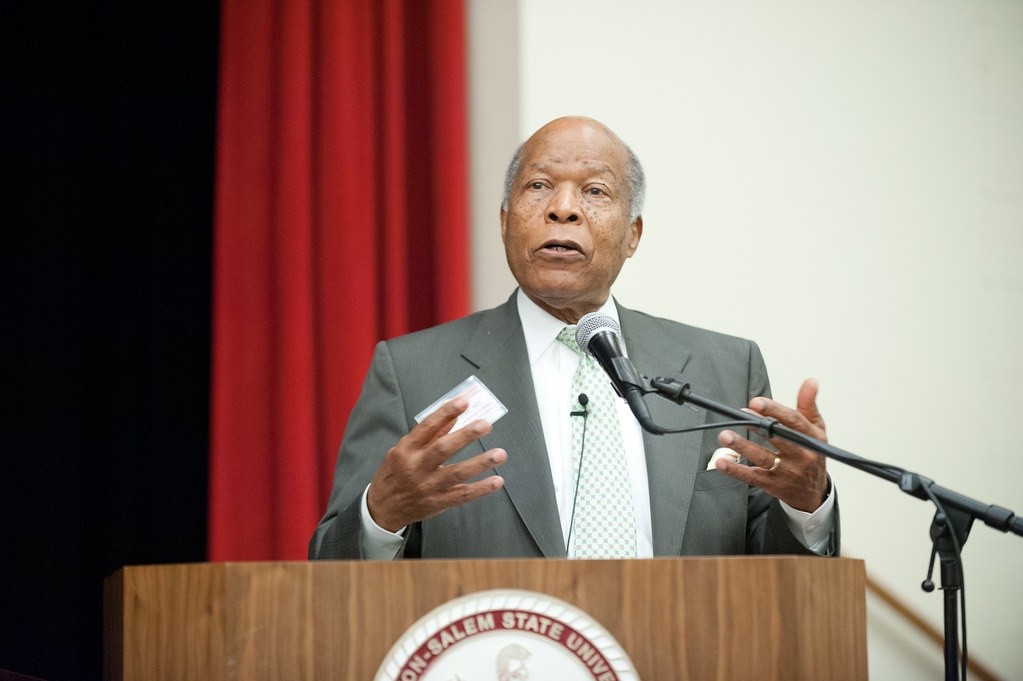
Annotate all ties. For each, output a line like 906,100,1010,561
557,325,637,560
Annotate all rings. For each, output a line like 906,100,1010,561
768,454,780,472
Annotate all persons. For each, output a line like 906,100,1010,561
309,117,842,559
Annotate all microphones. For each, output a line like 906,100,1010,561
576,312,664,434
570,393,588,417
